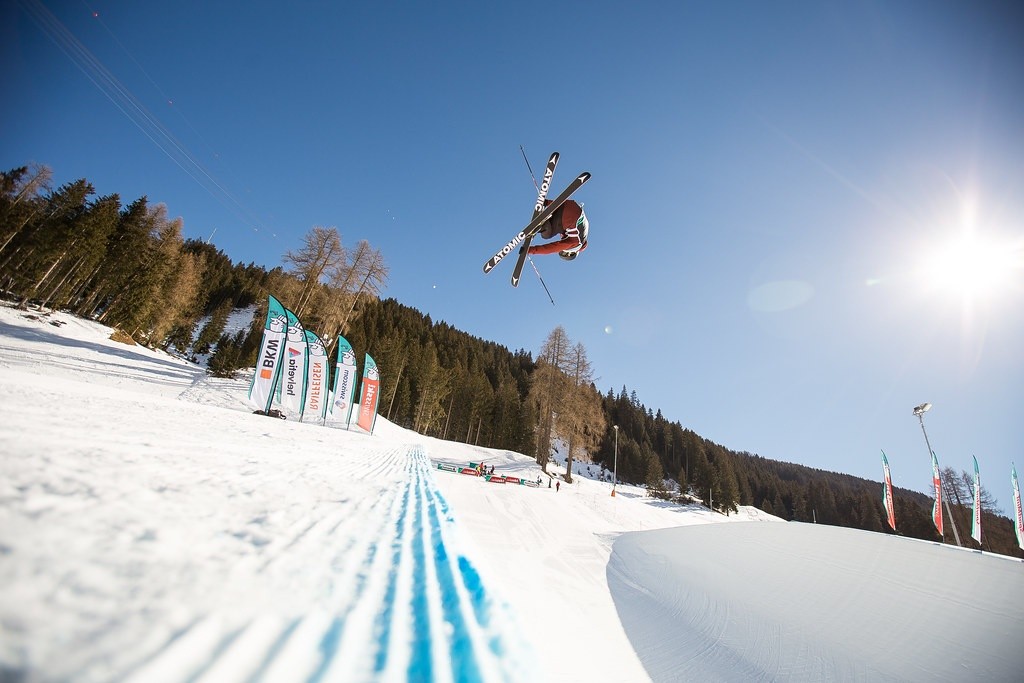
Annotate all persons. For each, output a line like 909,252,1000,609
519,200,589,260
537,475,560,492
476,462,495,477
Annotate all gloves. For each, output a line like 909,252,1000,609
519,247,524,254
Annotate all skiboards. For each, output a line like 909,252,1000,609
482,152,592,288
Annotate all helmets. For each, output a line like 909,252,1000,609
559,251,579,262
